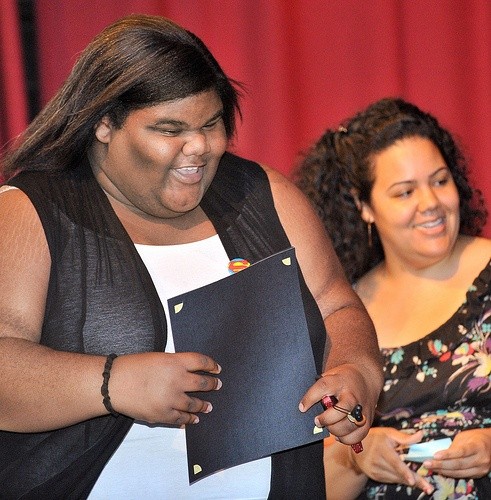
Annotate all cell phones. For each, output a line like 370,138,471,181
315,377,363,454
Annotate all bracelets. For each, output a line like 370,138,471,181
347,445,362,476
99,353,124,415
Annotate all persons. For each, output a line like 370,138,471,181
0,14,386,500
293,97,491,500
